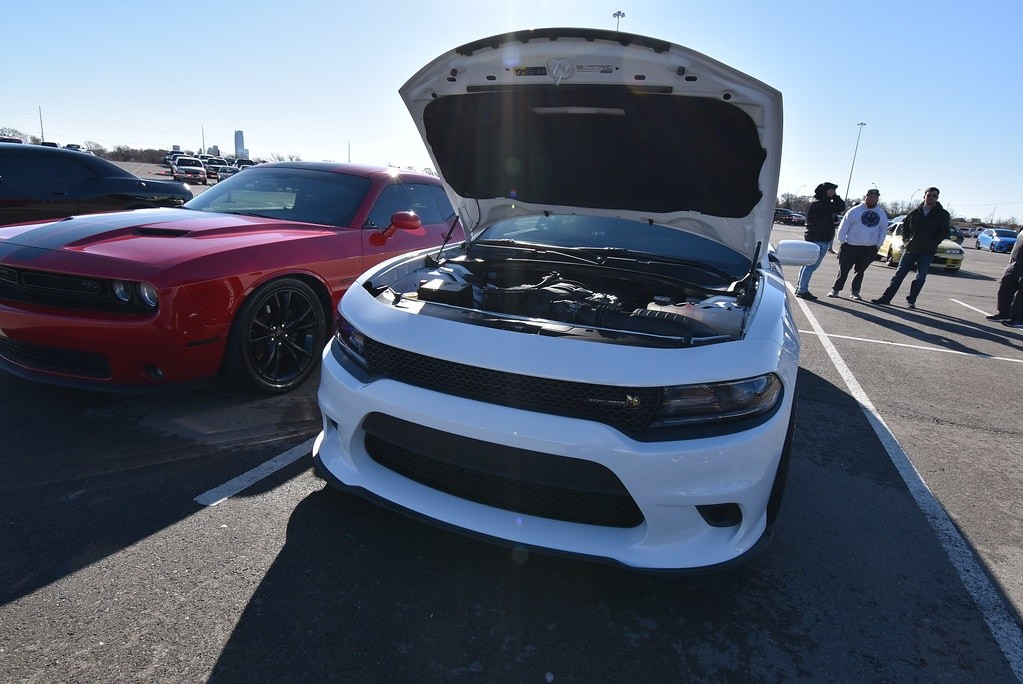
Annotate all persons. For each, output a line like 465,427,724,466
795,182,846,302
827,189,888,301
986,226,1023,328
871,187,952,308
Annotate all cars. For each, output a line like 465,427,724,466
774,209,807,225
0,138,22,143
215,155,255,170
64,144,86,152
0,159,467,399
164,150,191,173
41,142,60,148
975,229,1018,253
198,154,215,164
950,225,986,245
313,28,822,573
875,221,964,274
216,166,241,183
204,158,229,178
0,143,194,226
171,156,208,186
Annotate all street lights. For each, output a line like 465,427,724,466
845,122,866,203
612,11,625,31
909,189,921,206
872,182,877,189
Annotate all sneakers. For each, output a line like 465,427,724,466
985,313,1012,322
849,292,862,300
1000,319,1023,328
827,289,839,298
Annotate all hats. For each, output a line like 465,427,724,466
866,189,881,196
813,182,838,200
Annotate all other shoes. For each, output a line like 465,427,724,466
794,289,818,300
906,301,915,308
871,296,890,305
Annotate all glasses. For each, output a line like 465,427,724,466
927,194,938,197
